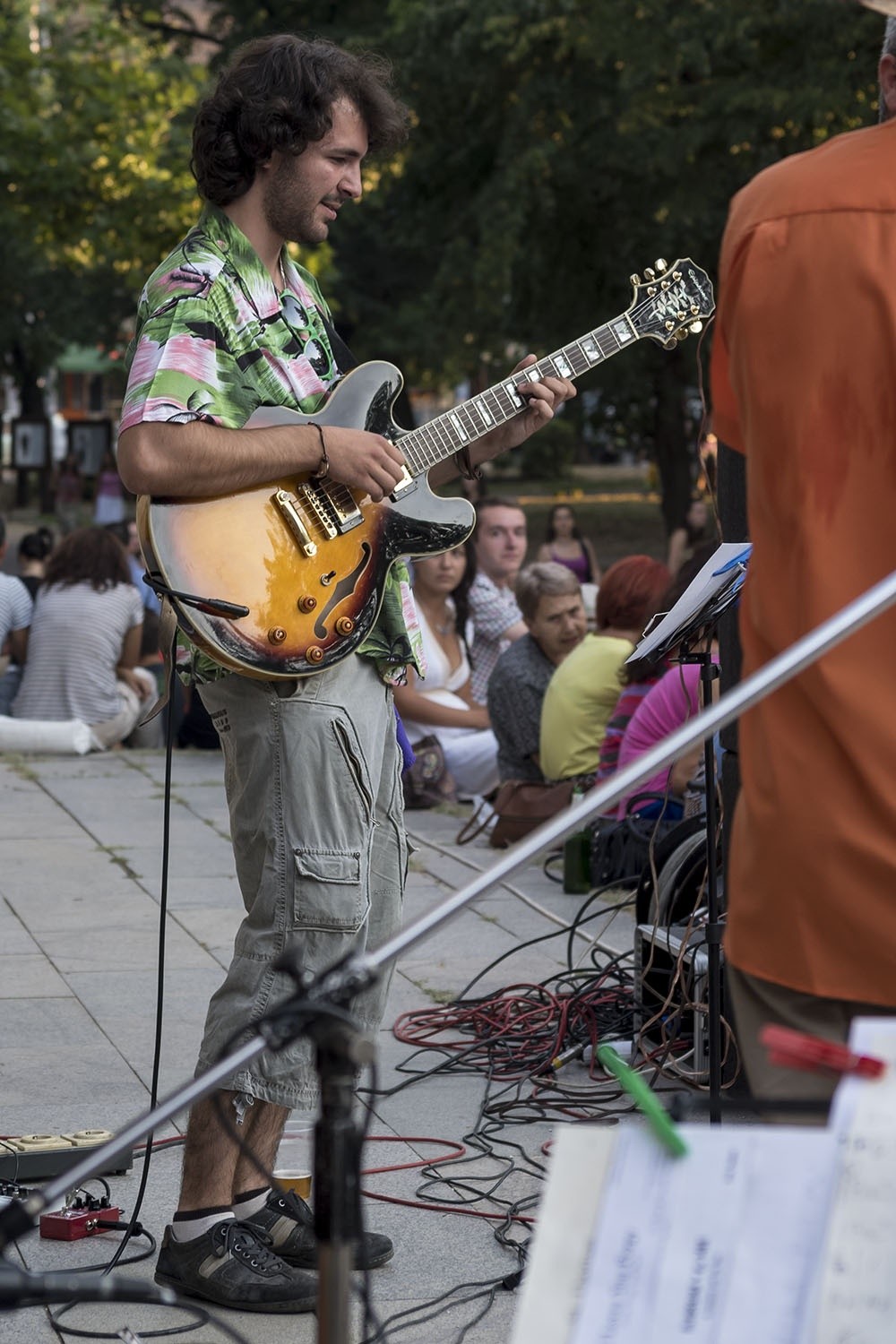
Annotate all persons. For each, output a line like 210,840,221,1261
397,497,721,888
115,37,577,1314
0,516,222,750
705,12,896,1122
11,441,129,523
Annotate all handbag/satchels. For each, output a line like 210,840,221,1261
404,717,460,809
457,781,574,849
591,792,723,890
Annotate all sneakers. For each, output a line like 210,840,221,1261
240,1187,395,1271
154,1218,320,1315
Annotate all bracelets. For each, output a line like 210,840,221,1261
305,419,331,487
455,445,482,485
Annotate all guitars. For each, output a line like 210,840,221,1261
135,258,716,682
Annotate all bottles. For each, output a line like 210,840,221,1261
564,783,590,894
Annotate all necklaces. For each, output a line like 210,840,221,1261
273,253,287,293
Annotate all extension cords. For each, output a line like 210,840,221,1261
0,1129,132,1182
